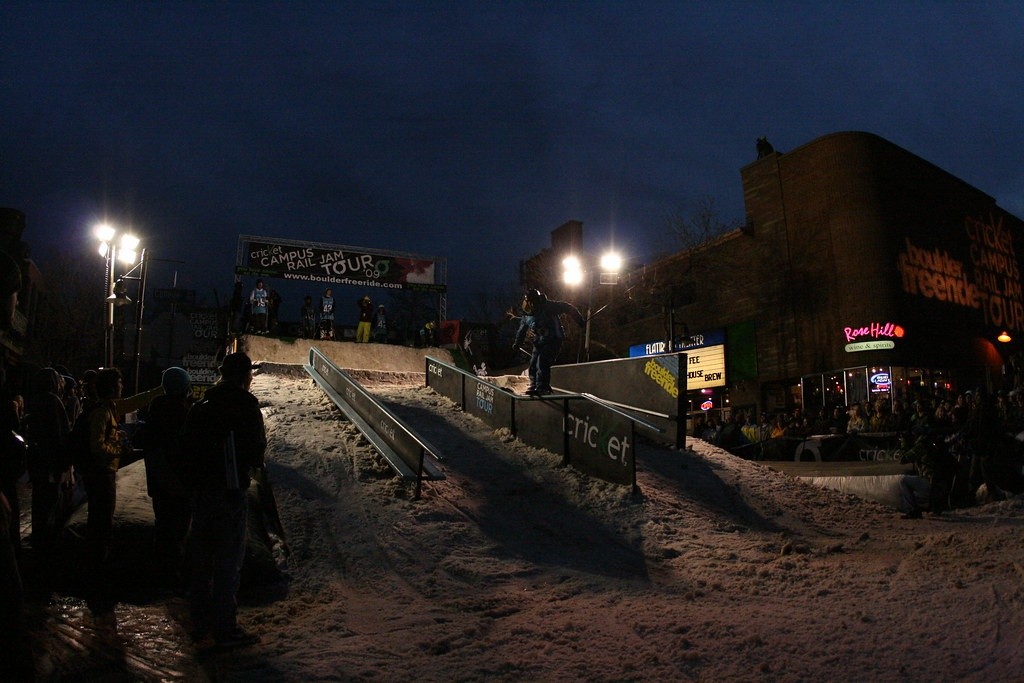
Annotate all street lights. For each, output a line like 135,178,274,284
104,252,150,397
668,298,695,353
560,251,623,361
93,222,143,366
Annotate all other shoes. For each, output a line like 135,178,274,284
216,629,260,649
902,511,922,519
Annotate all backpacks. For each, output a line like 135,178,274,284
66,404,101,463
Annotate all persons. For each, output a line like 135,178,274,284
319,287,337,340
375,308,391,344
507,288,586,395
355,295,374,343
301,295,317,338
249,279,268,335
692,386,1024,518
0,353,268,651
